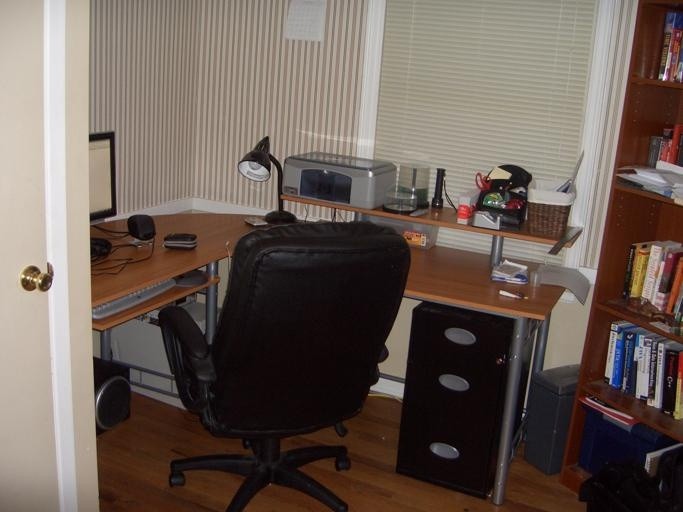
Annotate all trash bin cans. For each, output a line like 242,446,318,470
524,364,582,475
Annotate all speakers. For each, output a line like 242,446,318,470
93,356,131,441
129,214,155,241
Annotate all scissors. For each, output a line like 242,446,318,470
475,172,492,191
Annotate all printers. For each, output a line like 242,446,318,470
283,151,397,210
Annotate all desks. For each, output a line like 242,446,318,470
90,214,228,399
229,195,582,506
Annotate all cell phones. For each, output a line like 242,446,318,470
245,217,268,226
163,233,198,250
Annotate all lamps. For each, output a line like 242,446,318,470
238,136,297,226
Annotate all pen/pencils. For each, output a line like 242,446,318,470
505,182,512,191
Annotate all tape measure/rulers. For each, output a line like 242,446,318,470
548,227,583,256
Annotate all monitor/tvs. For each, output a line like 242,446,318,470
89,131,117,258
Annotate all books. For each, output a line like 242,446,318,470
647,124,683,172
622,240,683,322
644,443,683,477
658,12,683,82
604,320,683,422
581,393,636,426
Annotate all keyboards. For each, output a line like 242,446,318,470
92,280,176,320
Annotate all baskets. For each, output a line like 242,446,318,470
527,180,576,240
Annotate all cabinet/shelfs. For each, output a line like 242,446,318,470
561,0,683,494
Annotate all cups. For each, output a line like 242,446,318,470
529,270,542,287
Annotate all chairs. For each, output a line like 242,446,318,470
158,221,410,512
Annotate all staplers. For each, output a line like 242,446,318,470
457,191,480,225
